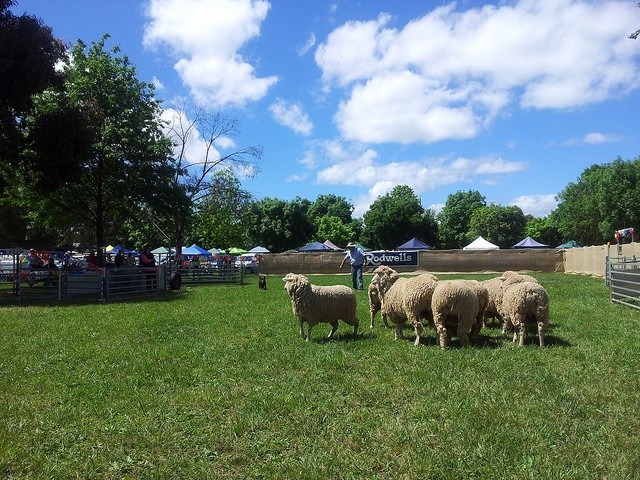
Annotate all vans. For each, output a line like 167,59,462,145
28,250,77,270
234,253,257,267
0,249,30,270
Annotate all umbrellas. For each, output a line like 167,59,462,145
106,245,137,254
463,235,499,251
299,241,334,252
217,248,226,254
181,243,211,261
220,247,248,256
397,237,431,249
249,245,270,255
151,246,174,265
323,240,345,252
354,240,372,251
207,248,220,254
555,240,587,248
129,250,141,256
512,236,550,248
102,245,114,253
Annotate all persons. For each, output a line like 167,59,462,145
615,229,621,245
45,258,58,269
87,251,97,270
29,248,40,266
192,255,199,261
126,252,136,267
338,242,366,290
114,249,124,268
139,246,155,274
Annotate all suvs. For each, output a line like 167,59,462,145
67,252,84,260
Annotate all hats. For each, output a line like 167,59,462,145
346,242,356,248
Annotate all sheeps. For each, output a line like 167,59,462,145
431,279,488,348
498,271,549,348
479,278,504,332
282,272,359,342
371,265,438,346
366,283,390,330
